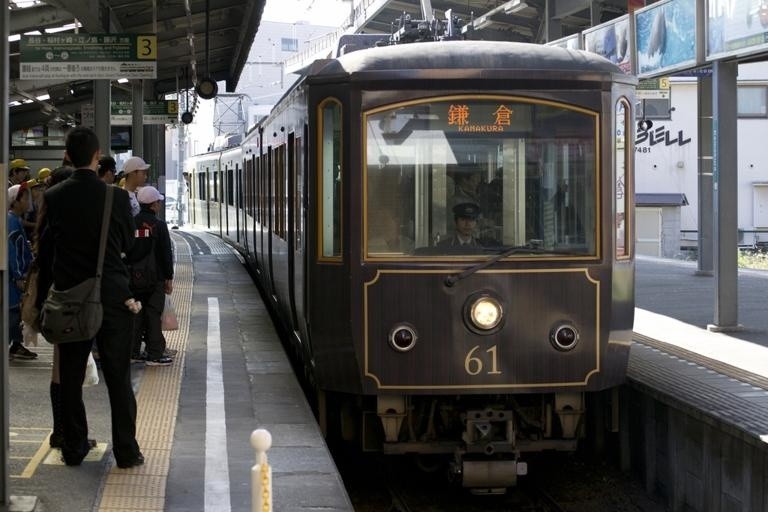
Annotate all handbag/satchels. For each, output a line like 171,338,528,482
36,278,103,344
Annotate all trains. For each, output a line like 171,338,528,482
181,6,642,498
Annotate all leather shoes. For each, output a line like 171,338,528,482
117,453,146,468
49,432,97,469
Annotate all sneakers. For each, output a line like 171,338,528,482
9,342,38,359
130,343,178,367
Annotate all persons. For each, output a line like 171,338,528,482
32,124,144,468
6,156,177,366
438,159,583,250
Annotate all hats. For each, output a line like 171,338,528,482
124,155,151,175
25,178,39,187
8,181,27,204
9,158,31,172
452,201,480,217
136,185,164,204
39,166,53,177
